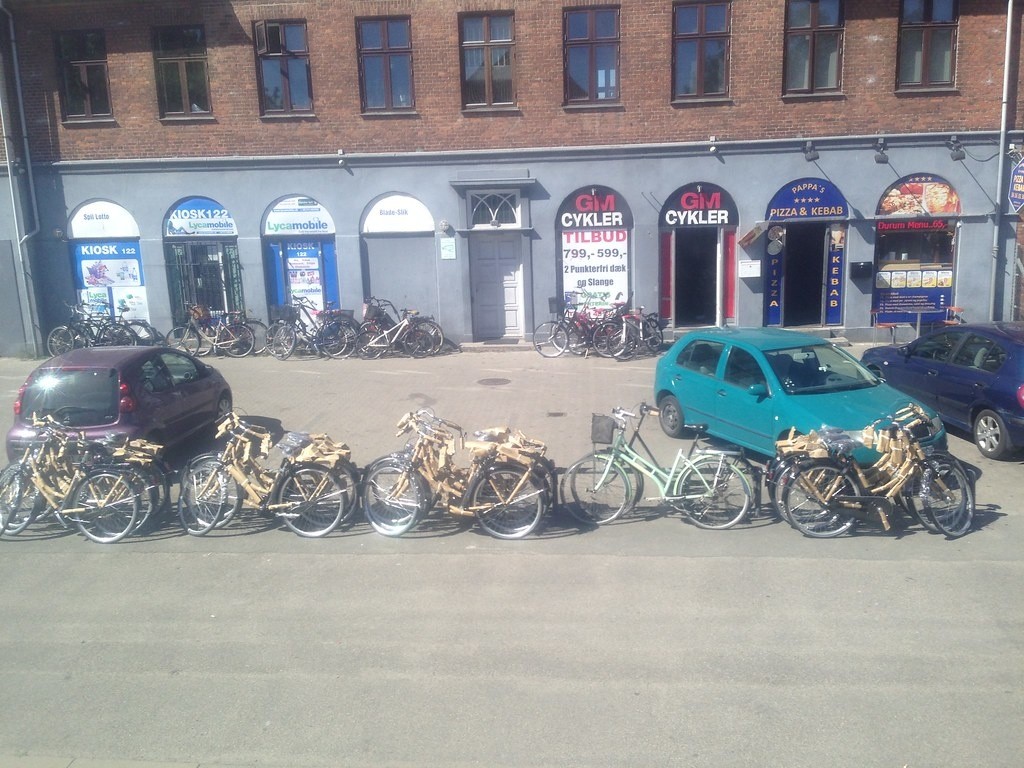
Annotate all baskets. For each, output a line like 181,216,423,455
280,305,296,322
340,310,354,321
549,297,563,313
176,311,188,323
368,307,379,320
591,413,615,444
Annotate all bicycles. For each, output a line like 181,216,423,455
531,289,667,363
361,408,555,541
47,298,155,358
559,402,751,530
763,401,976,539
178,407,358,538
0,404,172,545
164,296,444,360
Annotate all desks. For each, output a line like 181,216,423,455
901,308,943,344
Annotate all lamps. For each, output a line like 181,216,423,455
697,184,703,194
336,149,346,165
803,141,820,161
591,185,599,200
944,136,965,162
873,138,890,164
707,136,717,153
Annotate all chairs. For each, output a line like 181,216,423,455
773,354,794,378
974,348,999,372
690,345,718,366
870,309,896,348
939,306,964,328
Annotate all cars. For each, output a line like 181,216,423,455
5,345,233,473
862,320,1024,462
654,328,946,477
